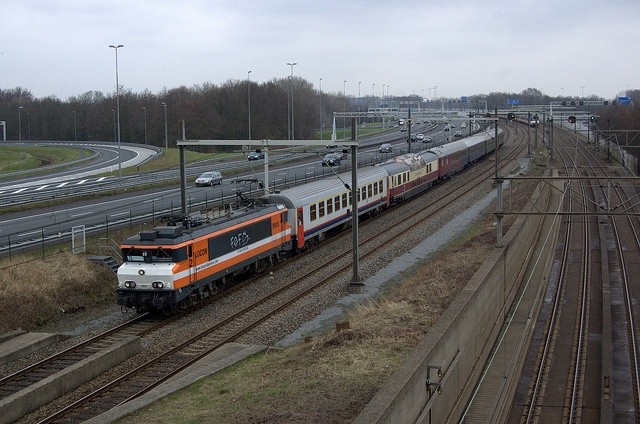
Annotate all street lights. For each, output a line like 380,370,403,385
248,71,252,150
372,84,375,127
412,84,439,101
162,102,169,157
358,81,361,125
286,61,298,141
344,80,348,138
27,112,32,141
111,109,117,142
287,76,292,148
73,110,78,141
383,83,386,130
18,105,23,171
109,43,126,182
320,78,324,140
142,106,148,144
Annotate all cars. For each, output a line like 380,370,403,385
379,143,393,153
454,130,462,137
423,136,432,143
322,153,341,166
248,151,264,161
407,133,418,143
417,133,424,140
195,171,222,187
443,119,467,131
334,149,348,160
395,118,429,132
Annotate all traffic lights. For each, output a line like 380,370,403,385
568,116,576,123
591,116,599,122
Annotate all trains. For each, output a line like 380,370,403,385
117,123,506,318
513,114,540,127
